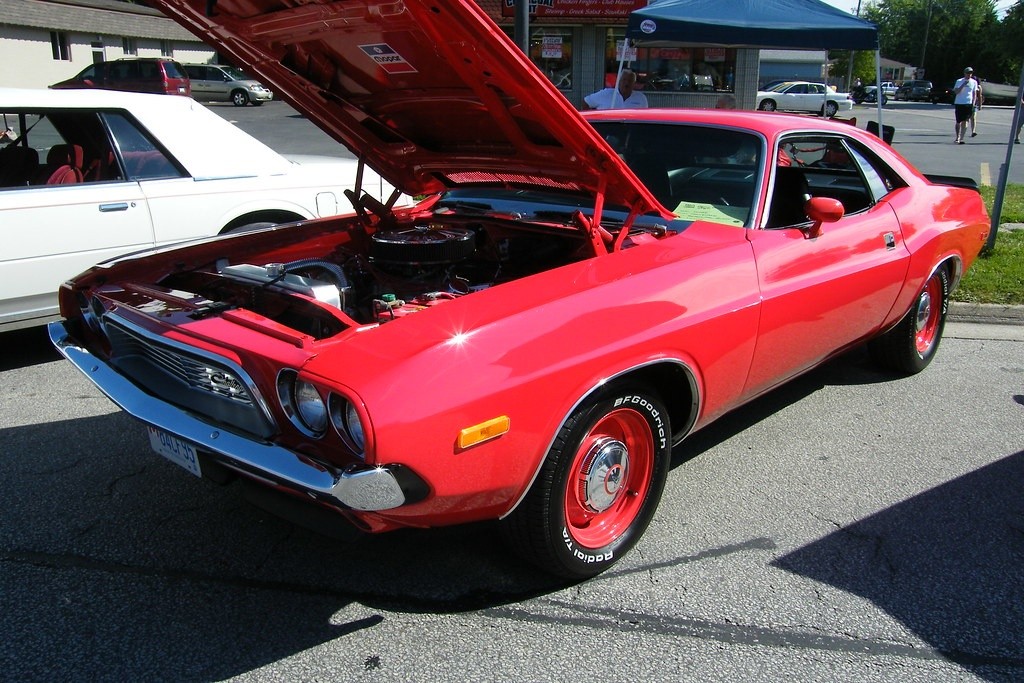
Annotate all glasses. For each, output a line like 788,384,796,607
624,67,637,73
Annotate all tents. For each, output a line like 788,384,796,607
611,1,886,144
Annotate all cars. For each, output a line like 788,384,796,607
0,85,417,329
759,77,838,92
756,81,854,117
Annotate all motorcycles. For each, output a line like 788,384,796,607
851,83,888,106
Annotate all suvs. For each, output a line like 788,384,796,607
49,56,193,99
894,80,938,104
174,63,275,107
48,2,992,581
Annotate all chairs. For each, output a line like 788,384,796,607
29,144,84,186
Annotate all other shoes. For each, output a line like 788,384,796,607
972,132,977,137
1015,138,1021,144
954,140,965,144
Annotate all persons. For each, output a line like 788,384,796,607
582,68,648,110
950,68,978,144
1013,90,1024,144
967,76,982,138
714,93,737,114
852,77,862,87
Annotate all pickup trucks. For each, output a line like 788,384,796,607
863,81,898,95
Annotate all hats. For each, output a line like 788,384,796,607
964,67,974,73
